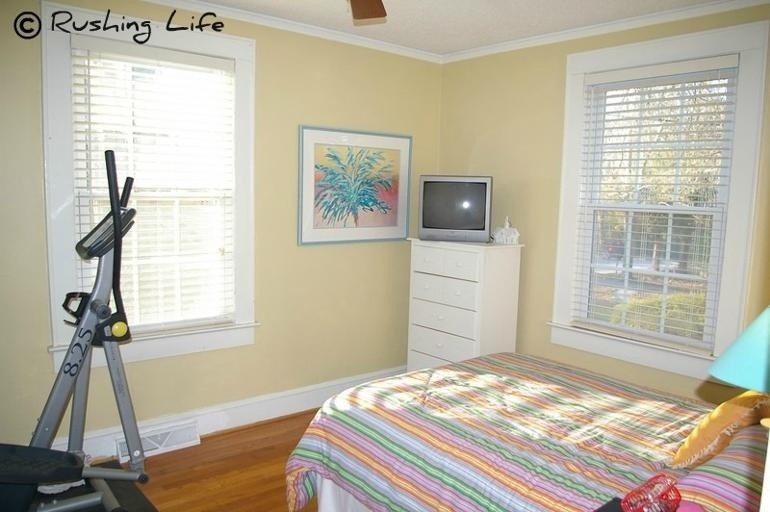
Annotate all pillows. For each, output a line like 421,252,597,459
672,390,770,470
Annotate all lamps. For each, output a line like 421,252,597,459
709,305,770,393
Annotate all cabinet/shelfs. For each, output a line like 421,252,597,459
404,238,526,375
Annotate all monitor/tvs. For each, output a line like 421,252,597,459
418,175,492,243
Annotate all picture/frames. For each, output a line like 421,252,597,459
296,124,414,246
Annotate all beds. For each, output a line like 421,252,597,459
316,350,770,511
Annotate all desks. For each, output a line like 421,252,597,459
34,460,159,512
